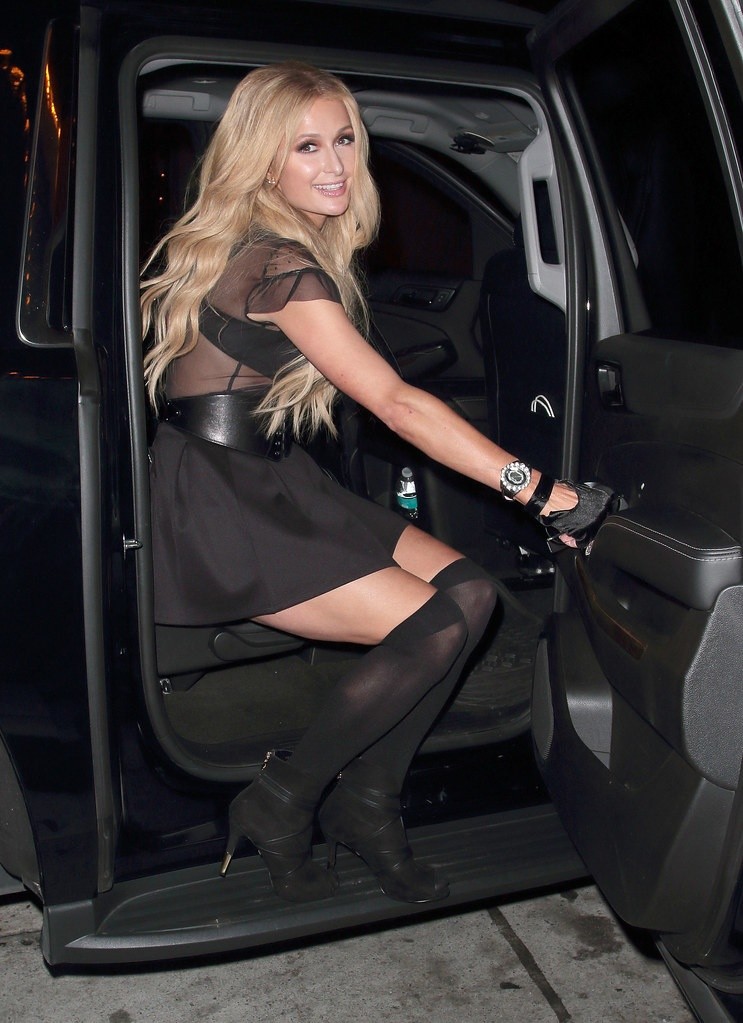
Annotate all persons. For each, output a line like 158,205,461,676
139,66,630,905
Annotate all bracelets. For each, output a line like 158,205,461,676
524,471,556,517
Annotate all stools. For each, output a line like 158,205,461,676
155,618,303,675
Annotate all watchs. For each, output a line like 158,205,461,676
499,461,532,501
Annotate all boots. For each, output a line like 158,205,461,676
220,749,334,903
318,756,450,904
